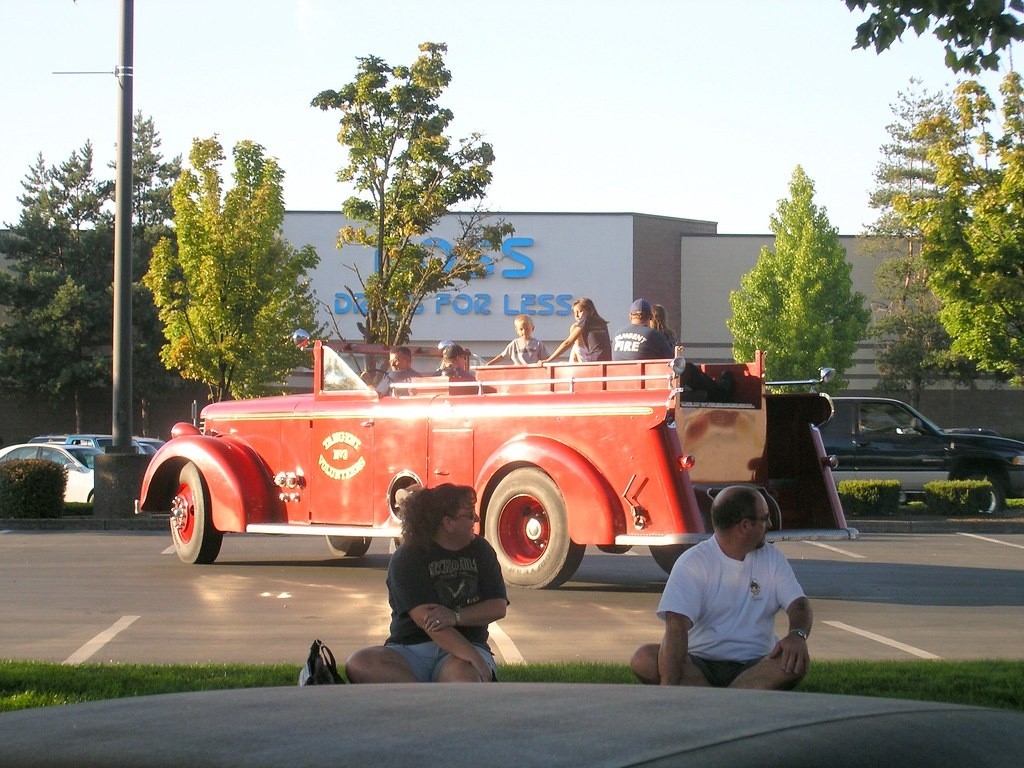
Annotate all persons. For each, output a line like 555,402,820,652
437,340,486,365
344,482,512,682
485,314,551,365
537,296,611,364
649,304,675,346
431,345,498,396
611,297,674,361
631,487,813,692
360,345,422,397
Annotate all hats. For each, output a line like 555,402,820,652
442,344,471,359
631,298,650,314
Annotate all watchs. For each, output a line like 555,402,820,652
790,629,807,640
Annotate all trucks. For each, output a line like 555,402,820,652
136,329,863,591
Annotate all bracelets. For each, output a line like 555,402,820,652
452,608,460,625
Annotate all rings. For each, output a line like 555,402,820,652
436,620,440,624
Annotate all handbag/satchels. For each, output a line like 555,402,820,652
299,639,346,685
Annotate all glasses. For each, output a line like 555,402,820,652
737,512,770,524
445,509,476,520
388,357,406,364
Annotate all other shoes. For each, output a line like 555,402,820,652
720,371,732,400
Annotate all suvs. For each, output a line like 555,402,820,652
823,395,1024,515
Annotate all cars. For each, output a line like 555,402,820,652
0,433,168,504
